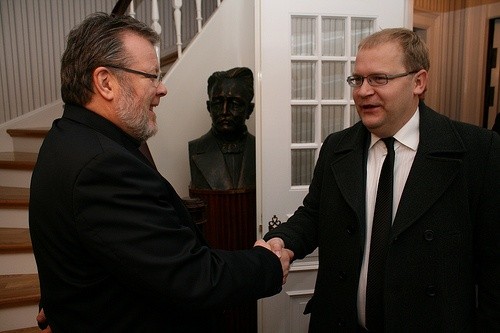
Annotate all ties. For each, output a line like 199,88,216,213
364,135,396,332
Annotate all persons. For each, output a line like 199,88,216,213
28,12,294,333
262,28,500,333
188,67,256,191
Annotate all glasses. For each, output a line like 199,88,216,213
348,71,417,87
96,64,163,86
